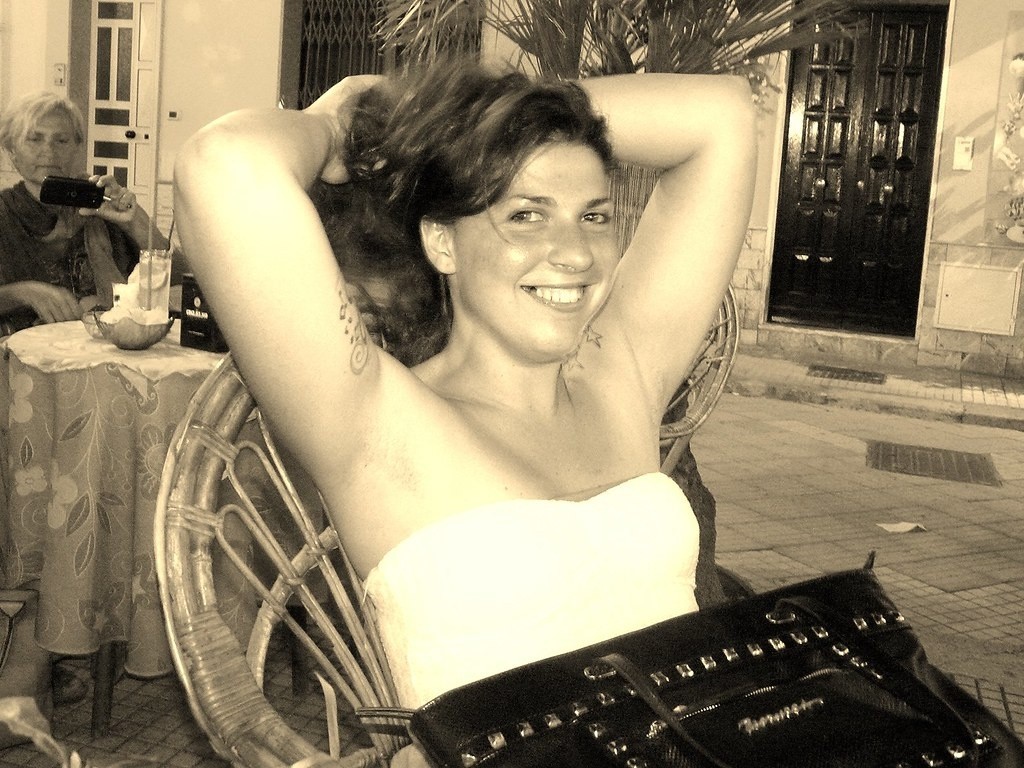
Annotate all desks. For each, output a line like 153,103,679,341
0,313,342,737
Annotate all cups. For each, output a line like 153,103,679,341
140,249,171,319
112,283,136,306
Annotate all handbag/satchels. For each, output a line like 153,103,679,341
410,551,1024,768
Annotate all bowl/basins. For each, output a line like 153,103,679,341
81,311,104,338
95,305,175,350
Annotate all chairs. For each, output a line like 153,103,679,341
659,283,740,476
153,280,411,768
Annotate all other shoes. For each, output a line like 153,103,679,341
49,663,88,703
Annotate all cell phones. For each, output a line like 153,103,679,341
40,176,106,209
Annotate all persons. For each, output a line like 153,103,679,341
172,69,760,768
0,92,186,336
